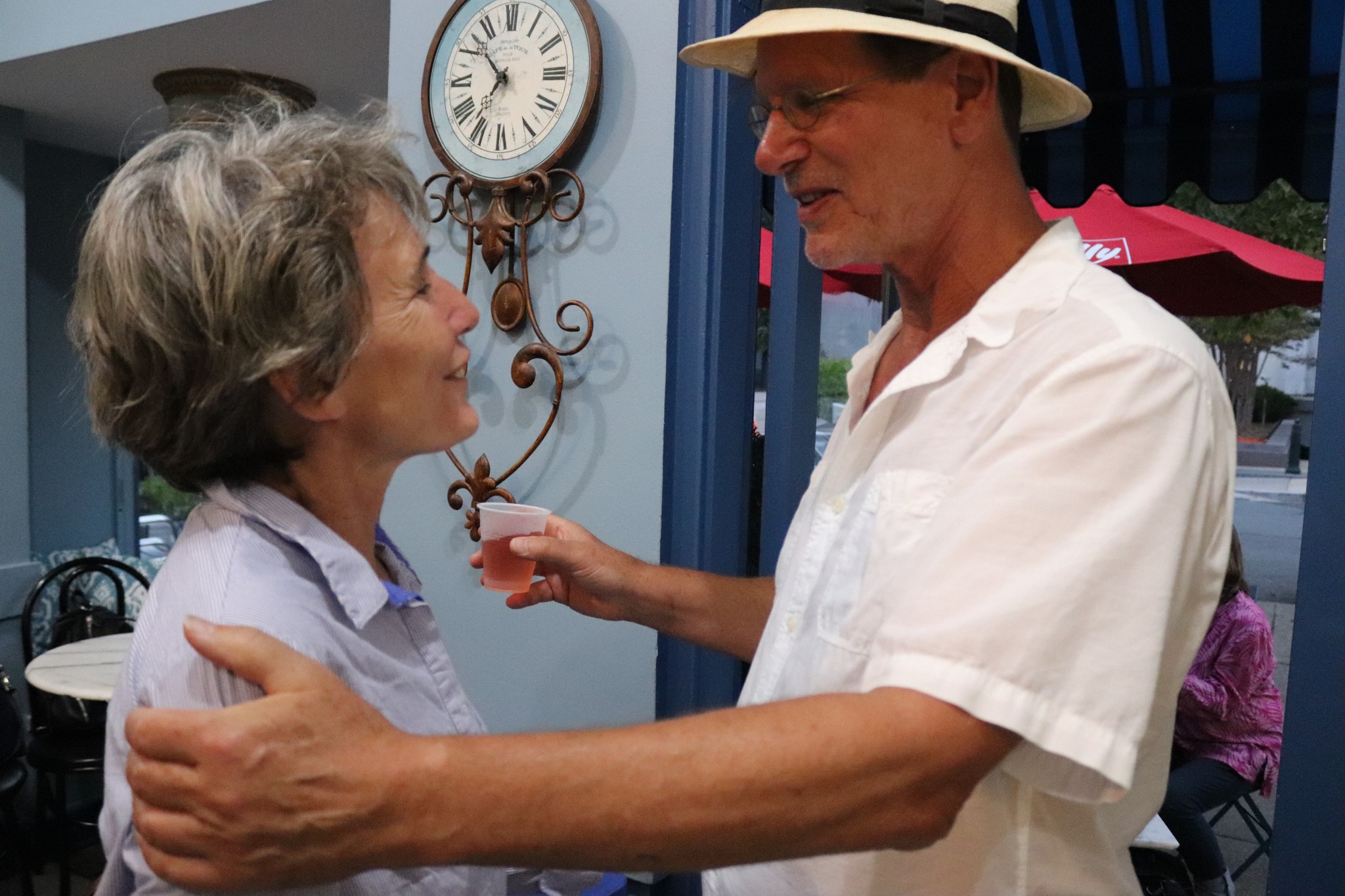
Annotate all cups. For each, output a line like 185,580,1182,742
476,502,553,593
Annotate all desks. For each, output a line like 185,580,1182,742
24,632,132,896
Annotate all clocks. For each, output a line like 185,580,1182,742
418,0,608,542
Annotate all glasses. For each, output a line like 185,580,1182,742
746,69,893,145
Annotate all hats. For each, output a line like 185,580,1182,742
678,0,1094,133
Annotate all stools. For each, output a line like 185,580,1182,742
1209,794,1273,879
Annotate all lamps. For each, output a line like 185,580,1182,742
150,67,316,124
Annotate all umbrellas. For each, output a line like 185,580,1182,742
734,182,1323,321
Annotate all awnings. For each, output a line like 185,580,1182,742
760,0,1345,206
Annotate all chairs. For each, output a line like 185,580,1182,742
21,557,150,896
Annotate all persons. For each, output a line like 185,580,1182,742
64,88,515,896
1158,523,1285,896
124,0,1237,896
